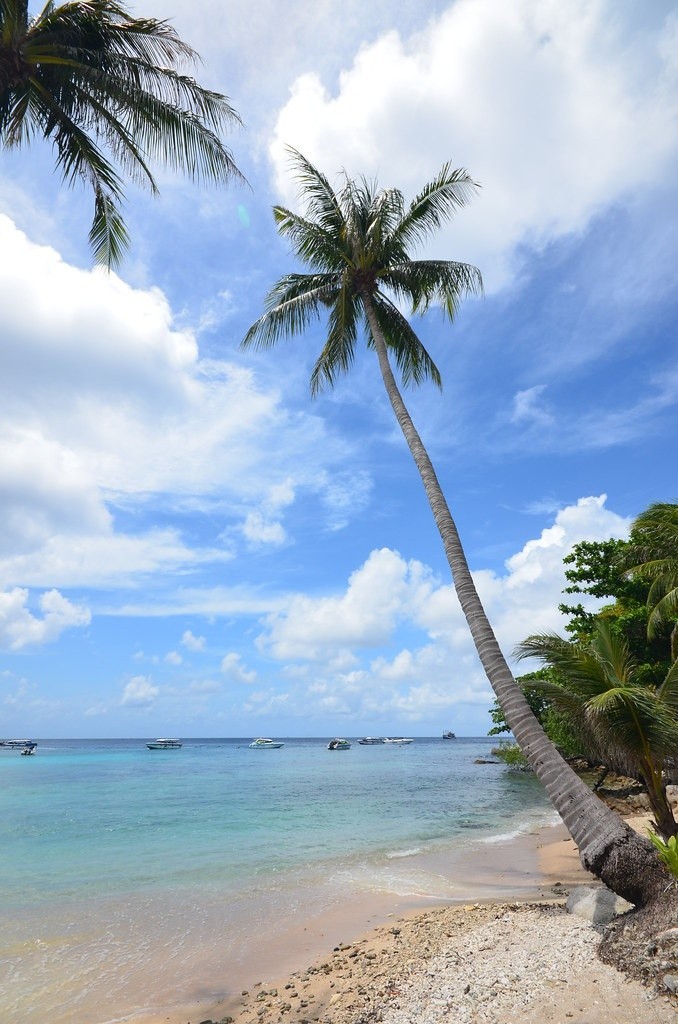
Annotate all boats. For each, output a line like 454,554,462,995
357,735,385,745
442,731,456,740
327,738,351,750
384,736,414,744
146,738,182,750
20,743,37,755
0,740,37,751
248,738,285,749
